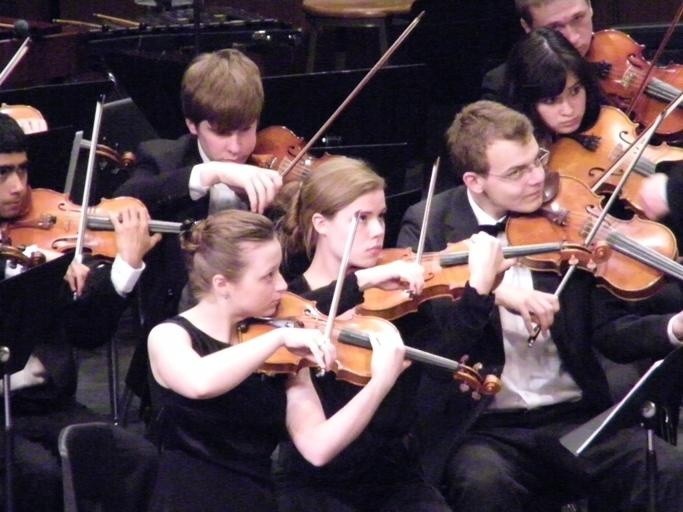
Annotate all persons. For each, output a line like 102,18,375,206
143,209,411,512
499,28,599,143
618,172,683,223
0,116,163,512
113,49,284,440
273,156,514,512
483,1,594,138
395,98,683,512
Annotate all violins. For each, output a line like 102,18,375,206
589,0,682,144
542,87,683,218
246,126,343,209
2,188,201,259
500,108,683,348
354,241,611,321
238,290,502,401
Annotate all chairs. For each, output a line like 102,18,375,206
57,419,160,510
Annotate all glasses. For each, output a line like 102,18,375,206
488,148,551,181
